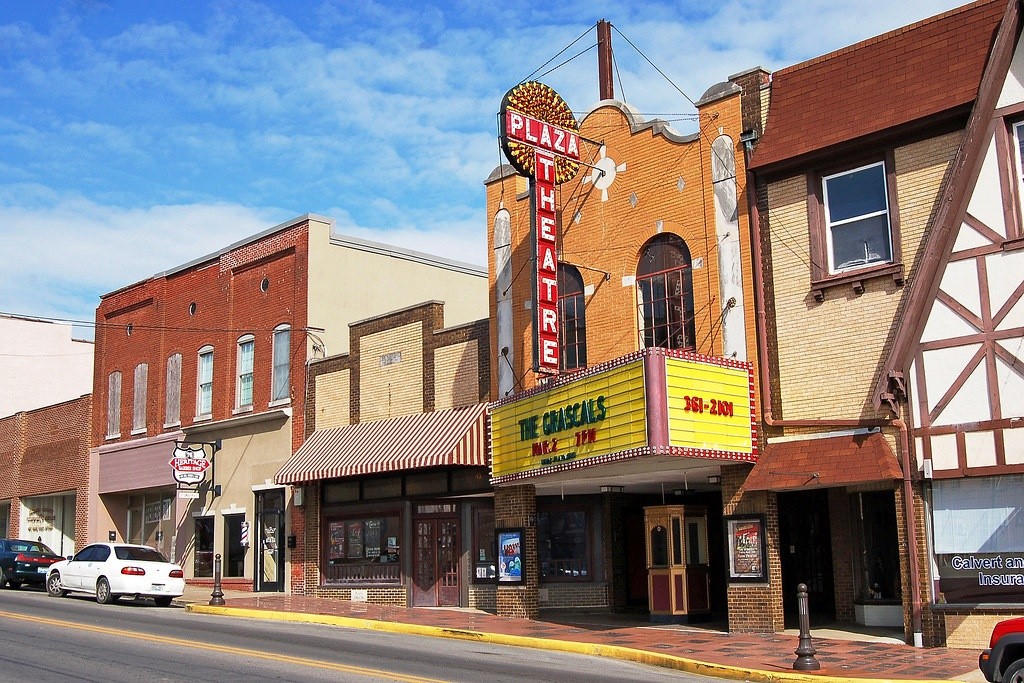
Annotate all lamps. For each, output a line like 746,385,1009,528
707,476,720,483
599,485,627,493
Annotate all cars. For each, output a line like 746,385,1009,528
46,543,186,608
978,617,1024,683
0,538,66,590
